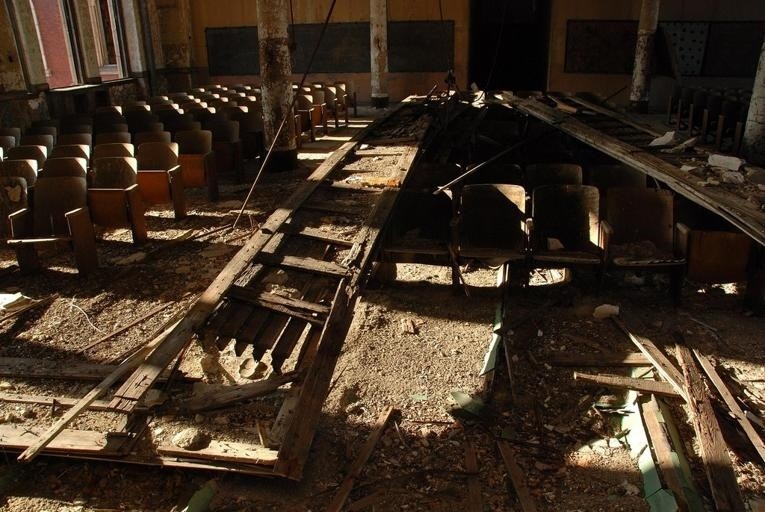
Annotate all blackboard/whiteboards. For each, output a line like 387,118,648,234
564,19,765,77
205,20,455,72
